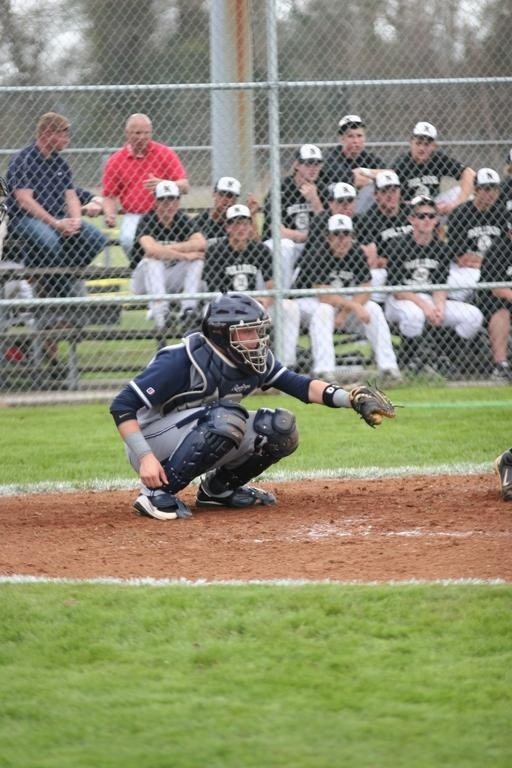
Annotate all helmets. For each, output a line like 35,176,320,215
201,293,272,377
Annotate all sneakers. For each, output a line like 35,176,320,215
310,371,337,385
424,356,453,381
491,361,511,380
196,484,255,507
156,306,200,328
139,490,187,512
381,370,405,389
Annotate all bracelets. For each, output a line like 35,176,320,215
123,431,151,463
322,383,342,408
333,388,352,408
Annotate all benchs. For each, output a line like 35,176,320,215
0,206,213,390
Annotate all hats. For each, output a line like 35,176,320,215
411,121,438,141
297,144,324,163
327,214,353,234
226,205,252,223
410,195,435,210
337,114,364,135
374,170,401,191
331,182,360,201
215,176,241,196
155,180,179,199
474,168,503,188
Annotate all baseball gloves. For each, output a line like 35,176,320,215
348,385,396,425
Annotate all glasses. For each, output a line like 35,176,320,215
417,213,435,219
337,198,354,203
333,230,349,236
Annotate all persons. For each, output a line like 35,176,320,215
110,294,396,520
201,204,299,374
101,113,191,260
261,116,512,386
193,176,258,245
4,111,108,326
75,186,102,218
127,182,206,329
0,177,47,373
